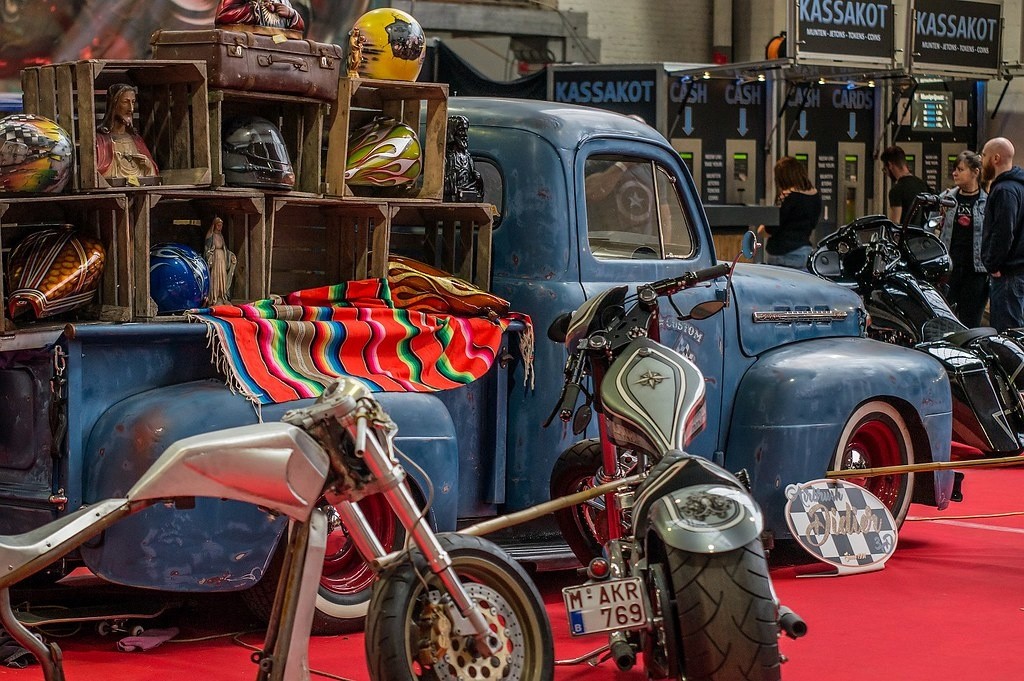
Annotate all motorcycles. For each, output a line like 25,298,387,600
0,376,556,680
546,264,807,681
805,187,1024,465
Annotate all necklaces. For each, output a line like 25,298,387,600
960,189,980,194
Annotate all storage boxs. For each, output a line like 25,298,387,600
0,55,496,340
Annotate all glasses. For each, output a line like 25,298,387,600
881,164,889,173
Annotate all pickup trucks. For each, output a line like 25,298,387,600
0,92,965,633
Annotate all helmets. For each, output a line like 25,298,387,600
1,113,73,196
220,117,297,189
351,9,426,81
12,221,108,317
344,118,421,187
213,0,304,38
151,242,210,314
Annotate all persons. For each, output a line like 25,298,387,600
880,145,936,230
93,83,160,188
932,151,992,330
215,0,305,32
204,216,238,307
585,114,673,246
980,137,1024,392
756,156,822,273
444,115,484,196
